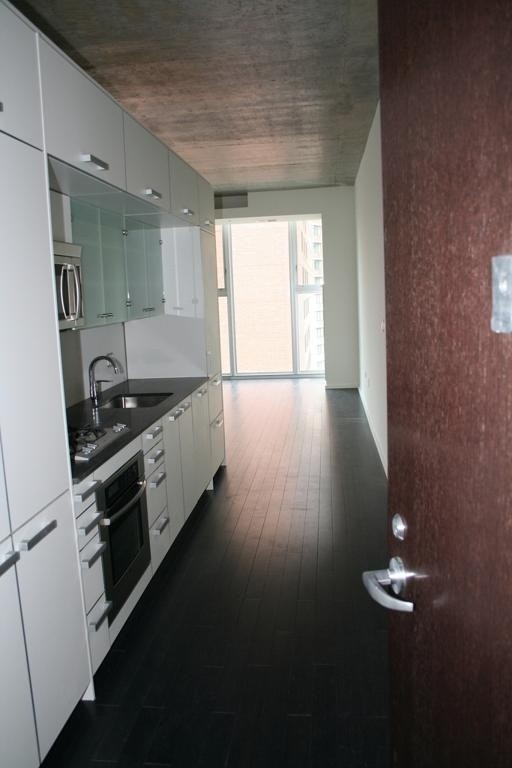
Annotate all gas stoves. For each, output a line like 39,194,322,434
67,425,130,462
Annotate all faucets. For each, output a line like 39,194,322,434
88,354,120,408
89,409,101,427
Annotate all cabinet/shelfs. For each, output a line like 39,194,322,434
73,438,155,677
192,372,225,505
138,393,193,574
2,130,53,233
2,7,44,151
70,197,164,329
122,227,221,379
135,156,216,233
0,435,88,768
37,35,126,201
92,113,170,216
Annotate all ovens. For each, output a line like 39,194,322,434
94,448,152,628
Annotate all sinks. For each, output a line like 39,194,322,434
98,390,175,412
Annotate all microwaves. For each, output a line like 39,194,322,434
53,255,84,326
